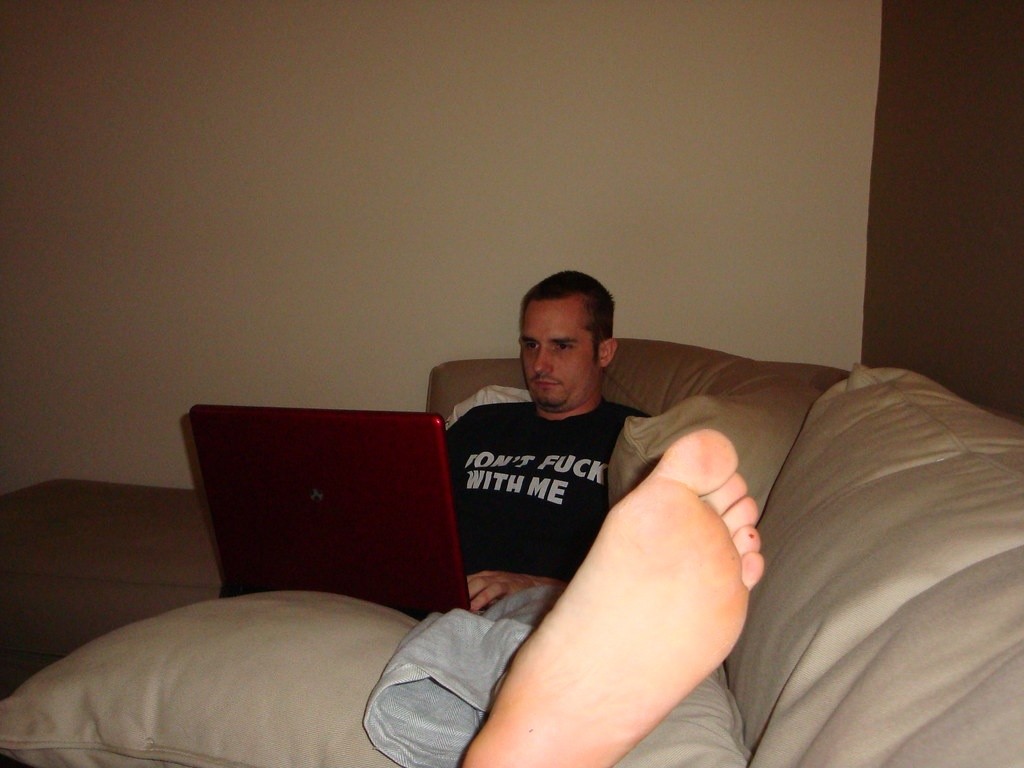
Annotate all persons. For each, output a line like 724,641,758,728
444,271,765,768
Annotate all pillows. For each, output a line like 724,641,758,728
0,589,420,768
613,663,751,768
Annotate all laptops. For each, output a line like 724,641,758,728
189,403,498,622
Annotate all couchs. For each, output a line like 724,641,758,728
0,337,1024,768
